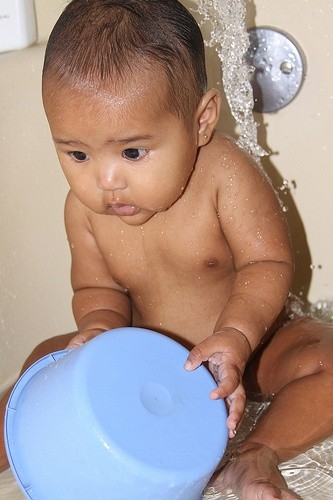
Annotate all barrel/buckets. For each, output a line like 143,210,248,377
3,327,228,500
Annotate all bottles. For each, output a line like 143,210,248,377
1,1,38,55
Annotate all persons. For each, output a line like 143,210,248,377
0,1,331,498
